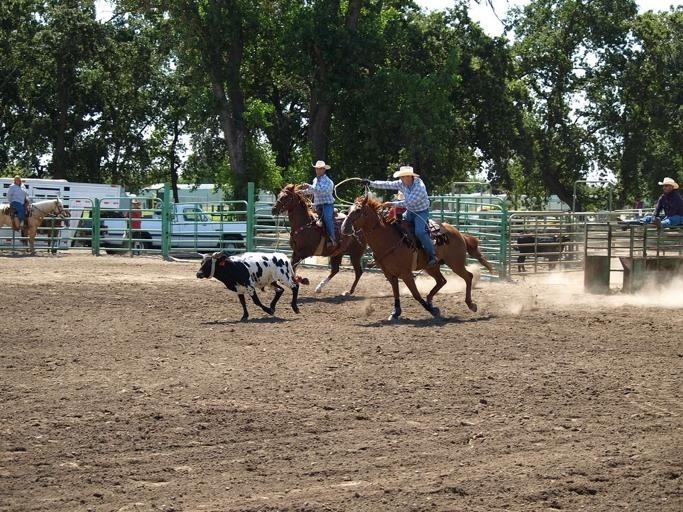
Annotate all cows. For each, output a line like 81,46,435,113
196,252,299,322
511,236,573,272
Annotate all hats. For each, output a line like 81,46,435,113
658,177,679,189
393,166,420,178
313,161,331,169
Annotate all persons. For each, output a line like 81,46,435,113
124,199,142,255
297,161,337,247
617,178,683,233
7,175,29,227
362,166,438,266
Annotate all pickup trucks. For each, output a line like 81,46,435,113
615,212,656,231
79,203,247,256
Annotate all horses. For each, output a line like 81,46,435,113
0,195,68,256
340,192,495,321
271,181,377,296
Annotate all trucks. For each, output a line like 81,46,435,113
0,177,129,248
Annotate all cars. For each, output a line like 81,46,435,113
253,202,276,227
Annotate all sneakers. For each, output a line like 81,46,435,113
616,218,627,231
327,236,336,242
428,256,437,265
656,217,661,231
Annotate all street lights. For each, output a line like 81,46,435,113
568,179,615,216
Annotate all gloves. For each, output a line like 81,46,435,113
361,179,370,185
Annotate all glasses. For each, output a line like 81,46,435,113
665,185,672,187
